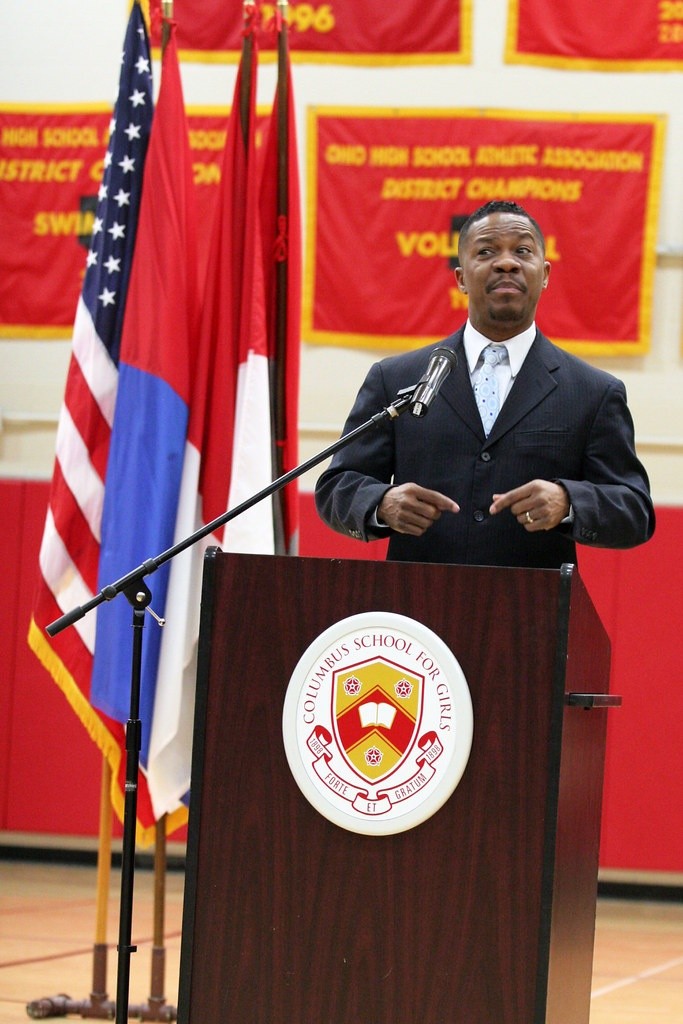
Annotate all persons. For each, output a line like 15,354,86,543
315,198,655,571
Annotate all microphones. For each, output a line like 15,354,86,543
409,347,458,419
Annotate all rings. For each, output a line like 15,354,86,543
526,511,533,523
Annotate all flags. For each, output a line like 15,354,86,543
23,0,322,863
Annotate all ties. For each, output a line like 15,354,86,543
471,345,511,439
